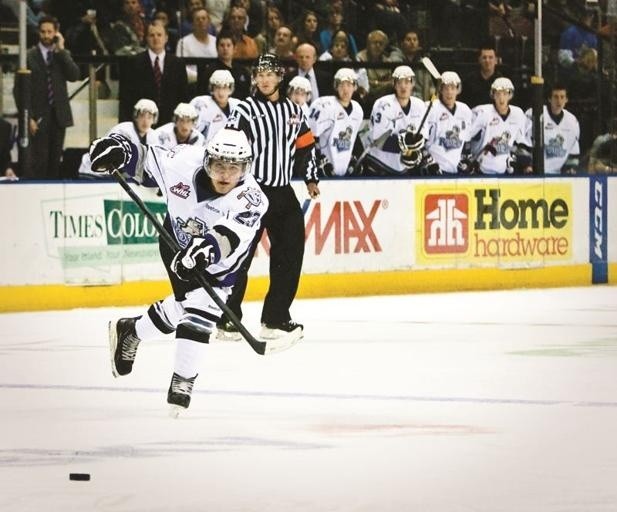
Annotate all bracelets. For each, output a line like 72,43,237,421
611,166,613,174
604,166,609,175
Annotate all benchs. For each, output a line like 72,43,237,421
1,74,119,148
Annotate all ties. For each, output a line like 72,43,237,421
45,51,55,106
154,56,162,89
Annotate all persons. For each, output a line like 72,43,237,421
305,68,364,177
524,84,581,175
0,119,19,181
74,69,241,183
464,44,526,107
90,129,271,418
222,52,320,340
14,14,79,181
424,71,476,177
69,0,433,129
462,0,540,43
363,65,427,178
471,76,526,177
541,0,616,82
587,133,617,176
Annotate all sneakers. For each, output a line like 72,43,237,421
114,315,143,378
167,372,198,410
216,318,242,333
259,321,303,332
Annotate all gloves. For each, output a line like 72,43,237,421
400,151,422,169
398,127,424,156
89,133,133,172
170,235,216,282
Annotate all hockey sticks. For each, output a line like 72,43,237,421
104,163,303,362
417,55,444,135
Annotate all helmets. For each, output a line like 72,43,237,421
203,124,252,183
439,72,461,96
491,78,515,100
251,55,284,86
209,70,235,95
133,98,159,124
334,68,358,91
288,76,312,102
392,66,415,89
174,103,199,129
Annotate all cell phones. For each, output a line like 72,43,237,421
53,36,58,43
